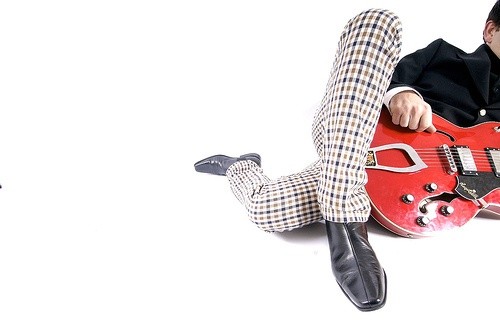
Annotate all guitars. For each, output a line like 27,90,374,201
361,107,500,240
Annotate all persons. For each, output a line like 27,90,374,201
194,0,500,312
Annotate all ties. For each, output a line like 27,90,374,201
493,78,500,94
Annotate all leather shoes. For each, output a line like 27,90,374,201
326,220,387,312
194,153,261,176
